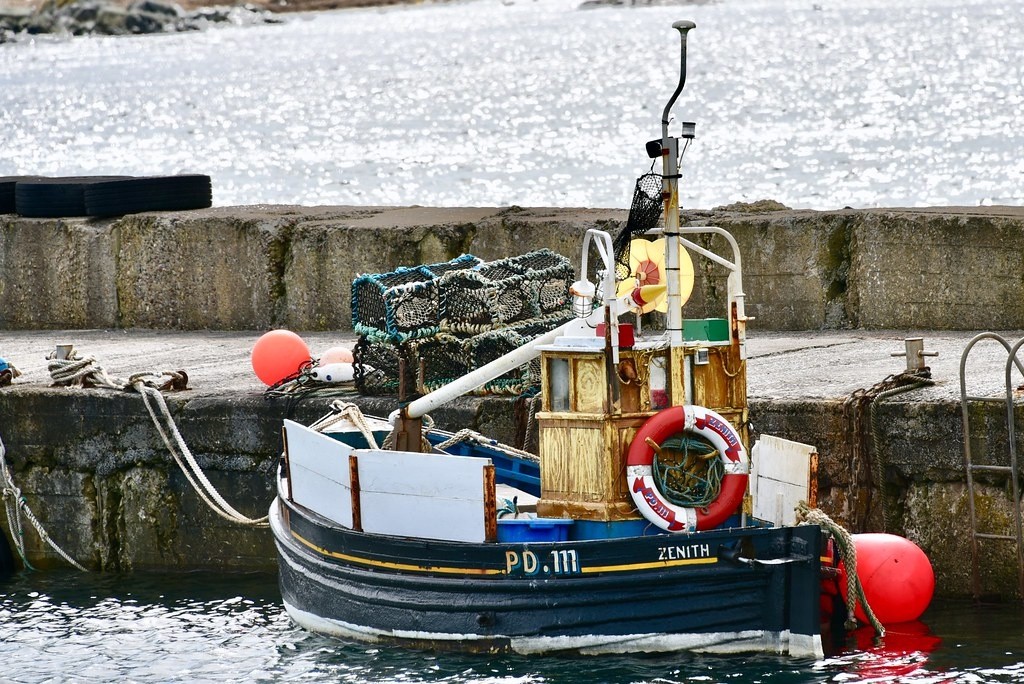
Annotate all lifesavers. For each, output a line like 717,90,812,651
624,404,751,533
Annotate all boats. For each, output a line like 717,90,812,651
268,20,828,669
